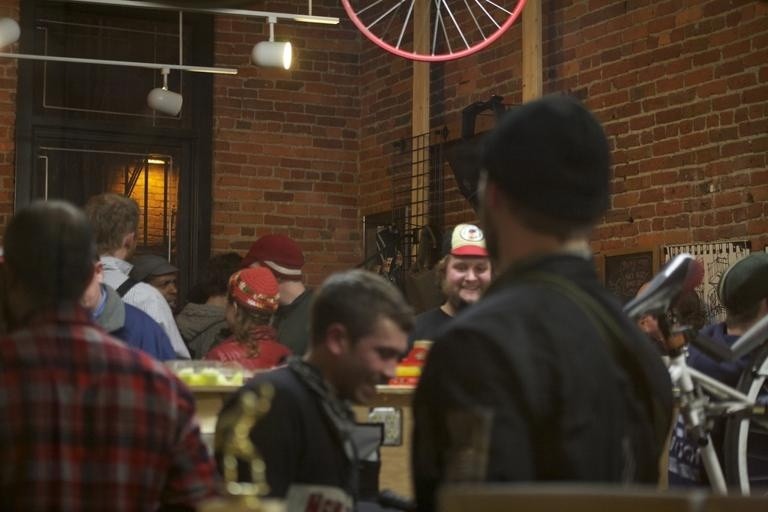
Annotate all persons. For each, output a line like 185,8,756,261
635,278,701,491
204,264,294,372
212,267,415,511
241,232,314,356
78,249,176,362
84,188,194,360
411,220,498,340
126,250,179,316
0,198,224,510
685,251,768,490
174,247,244,362
406,92,682,511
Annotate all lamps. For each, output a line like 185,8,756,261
0,16,20,50
250,40,293,71
148,88,183,116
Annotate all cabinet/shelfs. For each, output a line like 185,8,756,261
162,357,414,511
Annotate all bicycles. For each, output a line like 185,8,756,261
615,252,767,511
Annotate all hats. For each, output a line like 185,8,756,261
717,252,768,307
440,223,490,257
135,254,181,276
227,267,279,316
240,235,304,280
